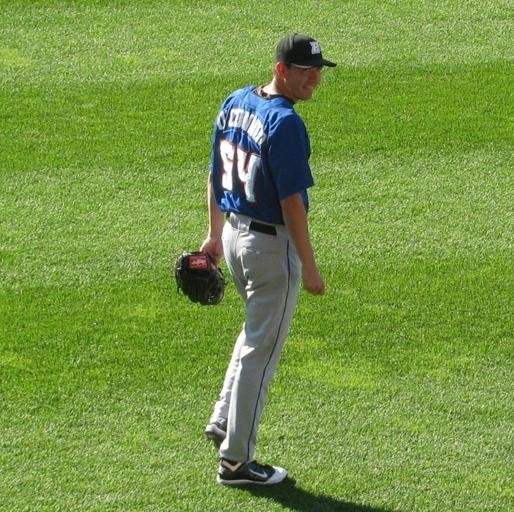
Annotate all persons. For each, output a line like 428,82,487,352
199,34,337,487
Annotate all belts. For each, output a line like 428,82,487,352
226,213,277,236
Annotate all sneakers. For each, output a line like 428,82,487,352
204,416,227,443
217,458,287,486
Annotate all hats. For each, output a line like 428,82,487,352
276,33,337,70
